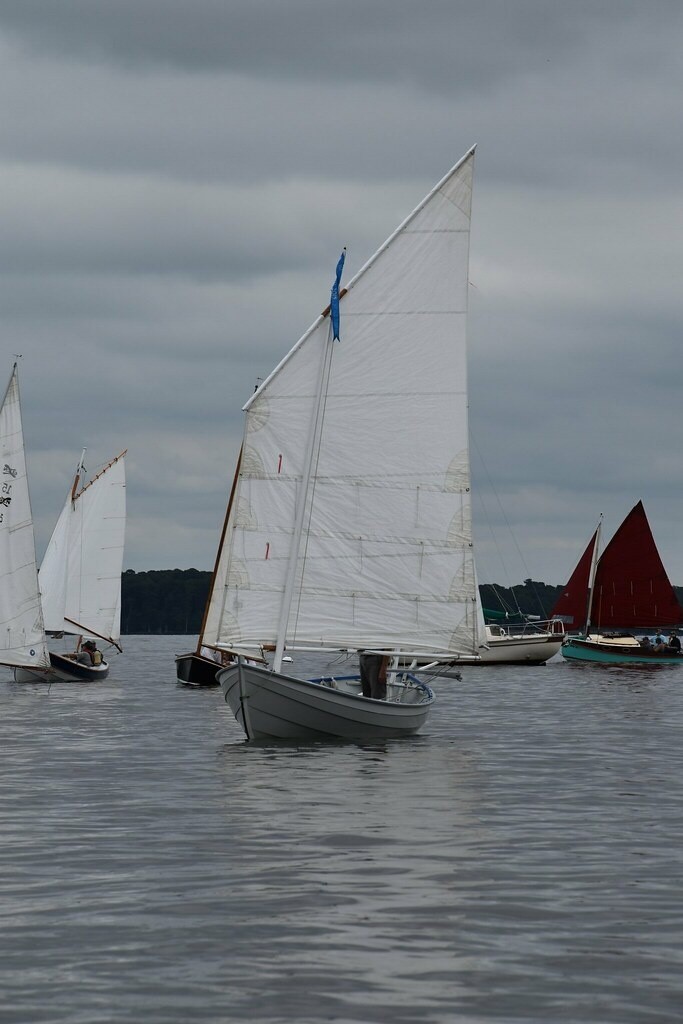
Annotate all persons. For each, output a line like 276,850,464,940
90,640,103,666
649,627,668,645
652,638,664,652
358,649,394,701
668,631,681,651
201,647,247,665
637,637,650,648
64,641,95,666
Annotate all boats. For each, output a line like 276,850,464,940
171,374,260,690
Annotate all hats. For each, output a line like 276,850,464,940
643,637,648,639
81,641,93,649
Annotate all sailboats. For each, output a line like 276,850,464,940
196,141,489,746
387,430,564,668
7,447,129,684
539,499,683,668
